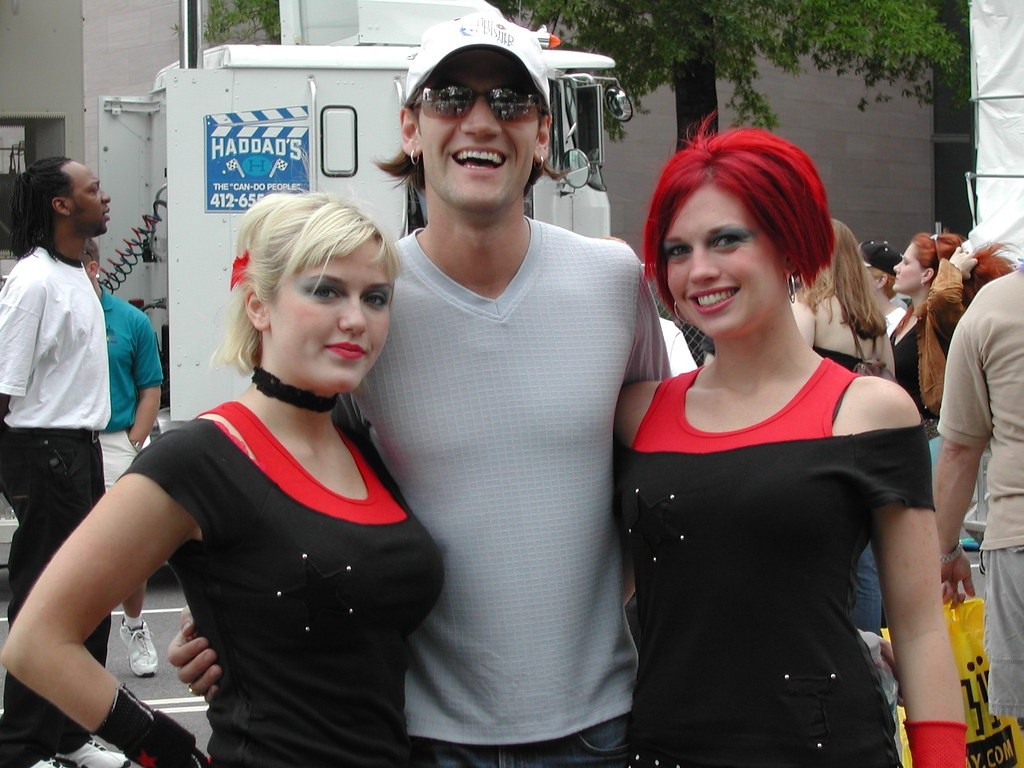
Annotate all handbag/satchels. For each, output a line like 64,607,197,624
881,597,1024,768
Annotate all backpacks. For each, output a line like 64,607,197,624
840,303,899,385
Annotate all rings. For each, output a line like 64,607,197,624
188,683,204,696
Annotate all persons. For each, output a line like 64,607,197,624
0,157,164,768
612,129,970,768
171,8,901,768
657,219,1024,768
1,191,446,768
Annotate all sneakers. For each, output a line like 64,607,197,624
30,735,131,768
119,617,159,678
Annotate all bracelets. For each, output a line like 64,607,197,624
940,539,962,564
124,709,196,768
130,441,142,450
905,719,970,768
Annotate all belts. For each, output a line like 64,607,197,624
7,428,100,444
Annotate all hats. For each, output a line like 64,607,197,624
406,11,550,114
859,239,903,277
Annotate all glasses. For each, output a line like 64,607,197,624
930,233,940,262
413,80,543,122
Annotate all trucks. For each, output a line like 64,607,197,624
149,0,636,422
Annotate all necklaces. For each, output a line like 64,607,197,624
251,361,340,413
